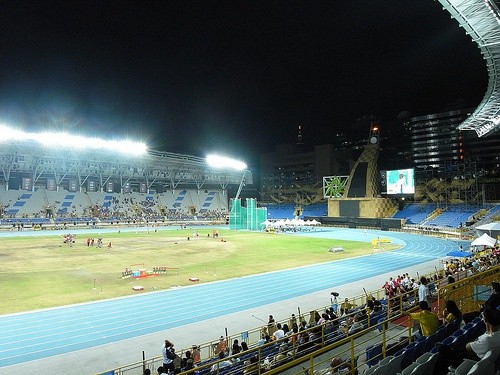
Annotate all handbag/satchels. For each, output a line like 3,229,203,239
165,348,177,359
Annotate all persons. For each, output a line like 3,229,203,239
396,302,439,337
143,271,420,374
417,277,436,311
2,189,229,248
438,300,461,325
478,282,500,311
437,307,500,371
415,213,500,294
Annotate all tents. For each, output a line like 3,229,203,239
260,218,323,233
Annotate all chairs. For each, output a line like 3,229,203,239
261,201,328,219
170,259,500,375
393,203,500,230
